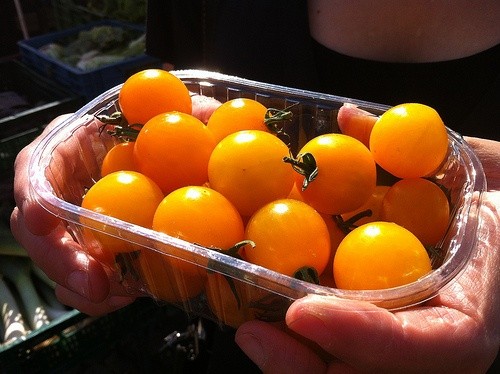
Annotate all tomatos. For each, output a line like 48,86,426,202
79,69,450,329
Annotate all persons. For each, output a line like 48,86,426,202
11,0,500,374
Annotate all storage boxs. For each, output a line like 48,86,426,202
0,295,258,374
0,0,150,226
